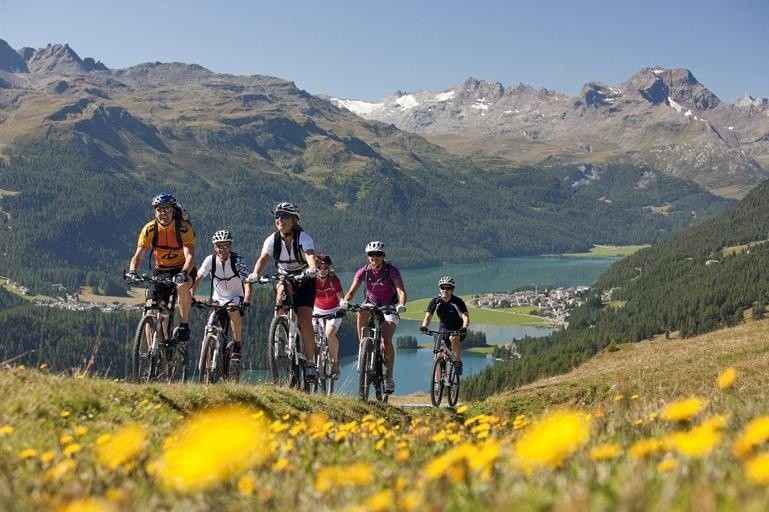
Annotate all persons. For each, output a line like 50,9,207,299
190,229,252,359
247,201,318,380
126,193,197,355
312,253,345,380
340,240,407,395
419,276,470,392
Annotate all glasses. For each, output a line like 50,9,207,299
156,207,173,212
368,252,383,256
276,214,288,219
441,287,451,290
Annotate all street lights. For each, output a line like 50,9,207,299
531,283,537,299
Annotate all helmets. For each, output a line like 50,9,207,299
365,241,386,253
271,202,300,219
152,193,176,207
212,230,233,244
315,254,331,266
438,277,456,287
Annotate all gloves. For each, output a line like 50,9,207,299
240,302,250,310
126,272,138,281
306,267,316,278
248,273,258,282
396,304,405,313
459,327,467,333
420,326,428,331
172,271,188,283
340,301,349,310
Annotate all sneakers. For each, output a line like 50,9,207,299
386,377,394,390
305,367,318,379
179,323,190,342
234,344,240,353
455,360,462,375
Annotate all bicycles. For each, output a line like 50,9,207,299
188,296,250,387
251,271,400,406
121,269,189,383
418,325,472,409
